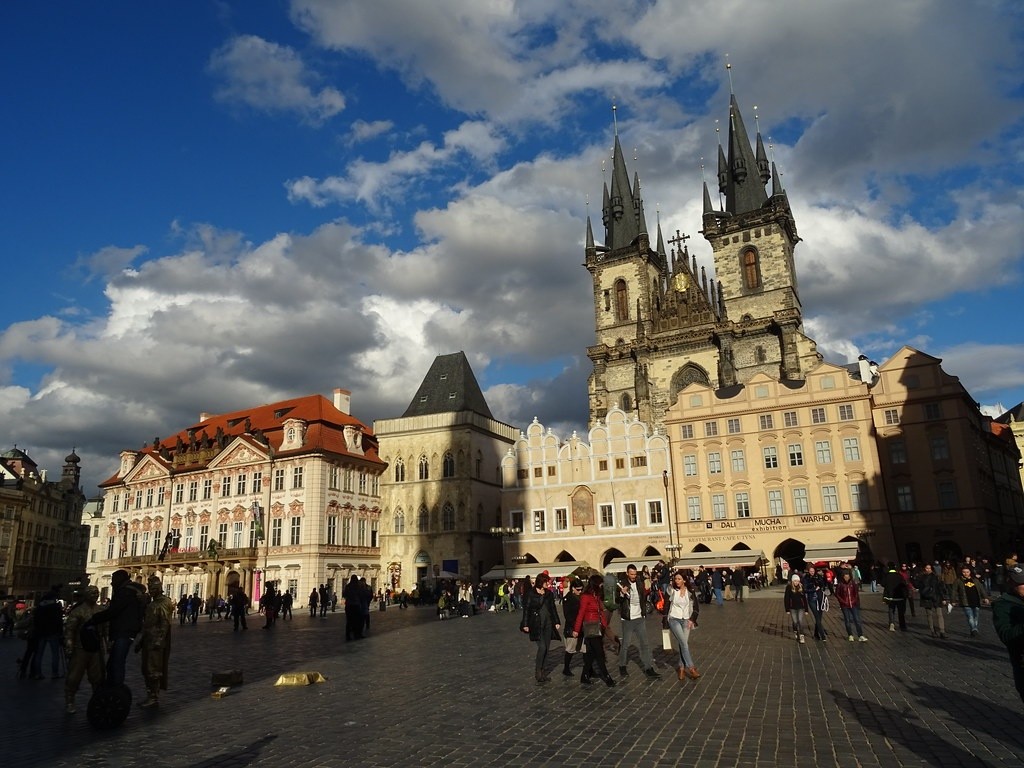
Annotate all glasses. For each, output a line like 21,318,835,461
574,588,583,591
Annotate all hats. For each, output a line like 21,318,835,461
792,574,800,581
1008,563,1024,584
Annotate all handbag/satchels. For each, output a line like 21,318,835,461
662,627,672,650
520,613,536,633
583,622,602,638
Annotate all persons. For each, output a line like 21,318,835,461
993,563,1024,703
177,575,425,641
774,561,879,644
86,570,146,729
437,575,570,621
63,574,172,714
20,590,63,679
0,600,18,638
519,564,701,687
881,552,1019,639
640,560,768,607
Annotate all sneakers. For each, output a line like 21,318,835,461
848,635,855,641
859,636,868,642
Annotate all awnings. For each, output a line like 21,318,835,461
803,541,860,562
672,549,770,588
605,555,669,575
481,560,603,580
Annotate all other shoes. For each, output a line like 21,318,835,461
581,675,594,684
795,634,799,640
940,632,949,638
822,635,827,641
563,669,574,676
619,666,629,676
972,630,977,636
543,677,551,681
606,675,617,687
814,634,820,639
538,679,544,684
889,622,895,631
932,632,938,638
35,674,46,680
678,669,686,679
51,674,63,679
689,669,702,679
647,668,661,678
800,634,805,643
